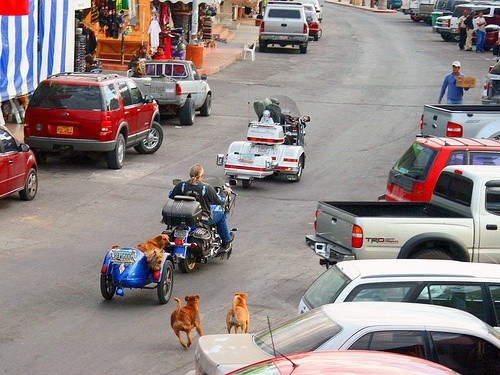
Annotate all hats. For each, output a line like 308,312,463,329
452,61,461,67
464,10,468,13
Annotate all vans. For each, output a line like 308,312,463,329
378,137,499,202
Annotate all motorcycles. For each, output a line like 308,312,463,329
162,179,238,274
216,97,309,188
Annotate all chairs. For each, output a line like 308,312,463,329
243,40,256,62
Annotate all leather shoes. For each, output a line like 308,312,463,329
222,232,235,246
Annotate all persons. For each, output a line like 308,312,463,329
458,9,470,49
172,38,187,61
78,48,102,72
134,50,147,78
474,11,486,54
128,46,153,79
464,11,475,51
85,54,102,71
153,45,166,60
438,62,469,106
170,164,235,245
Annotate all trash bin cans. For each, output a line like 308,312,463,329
431,12,443,33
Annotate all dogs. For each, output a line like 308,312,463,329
143,247,165,271
169,294,204,349
225,292,249,335
111,233,171,252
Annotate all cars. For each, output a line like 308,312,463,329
294,0,323,12
472,14,499,51
403,0,438,22
195,303,500,375
1,125,39,202
303,4,319,19
304,11,322,41
419,0,470,25
224,350,458,375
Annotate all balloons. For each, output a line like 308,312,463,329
205,10,212,16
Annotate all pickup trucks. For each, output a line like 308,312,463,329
305,165,500,263
420,103,500,137
126,60,213,126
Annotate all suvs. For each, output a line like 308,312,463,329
24,71,163,170
434,4,500,42
257,1,310,53
481,59,500,104
297,259,500,333
